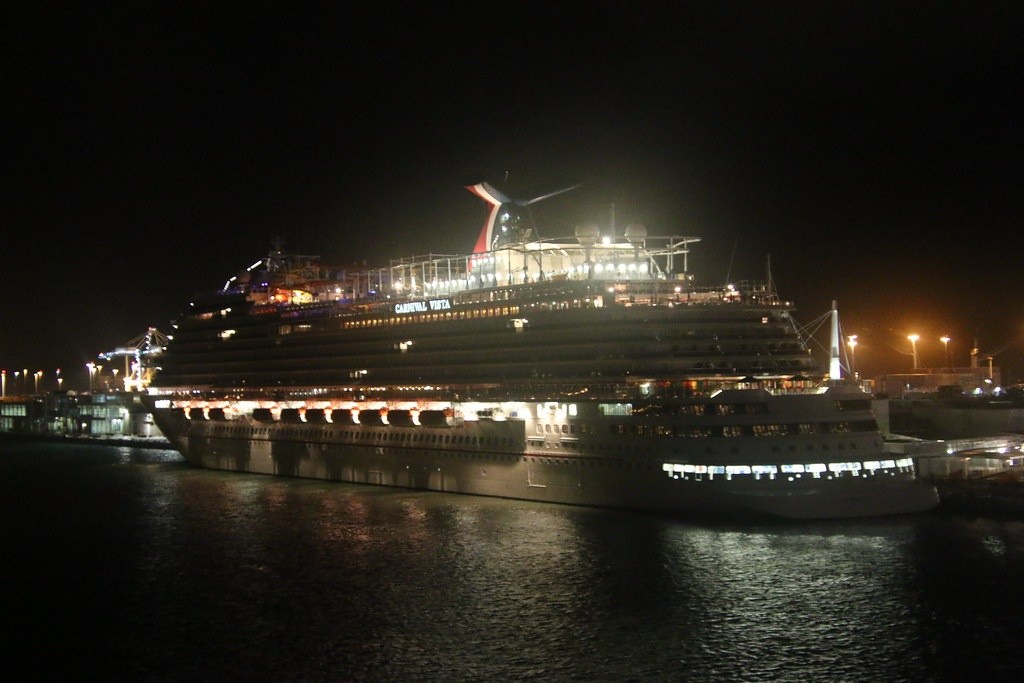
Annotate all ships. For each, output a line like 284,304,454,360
135,172,945,521
